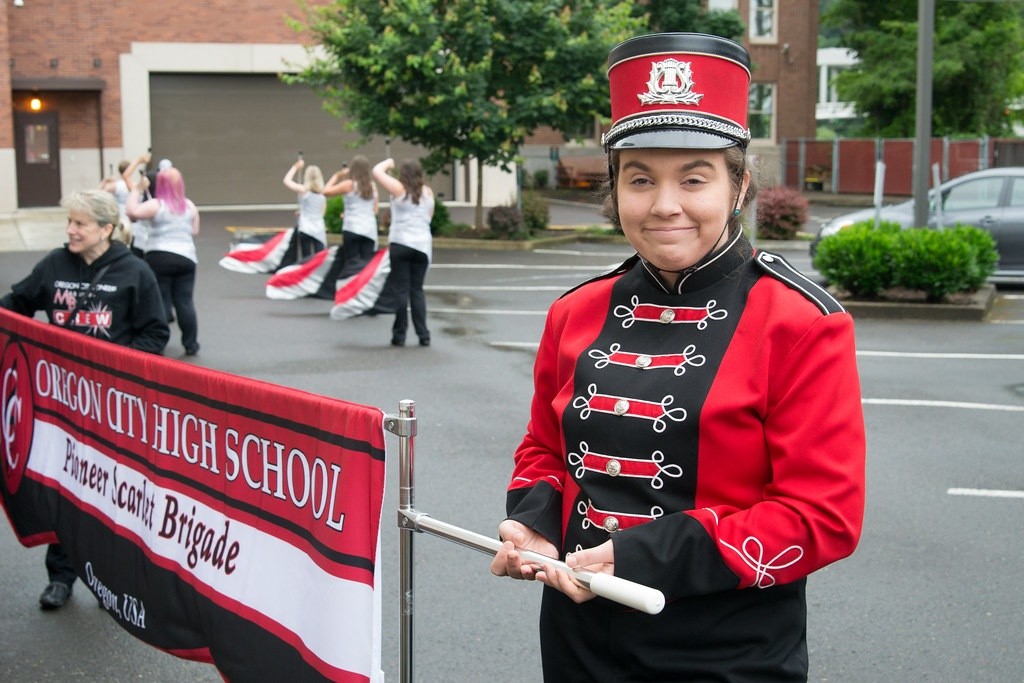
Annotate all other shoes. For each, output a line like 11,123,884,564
391,338,405,346
40,581,71,608
186,343,199,355
420,338,429,345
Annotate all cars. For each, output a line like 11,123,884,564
808,165,1024,285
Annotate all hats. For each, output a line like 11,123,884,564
601,33,752,149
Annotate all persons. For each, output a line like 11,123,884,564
0,190,171,608
283,160,328,262
323,155,379,256
490,33,865,683
99,152,201,355
373,159,435,346
